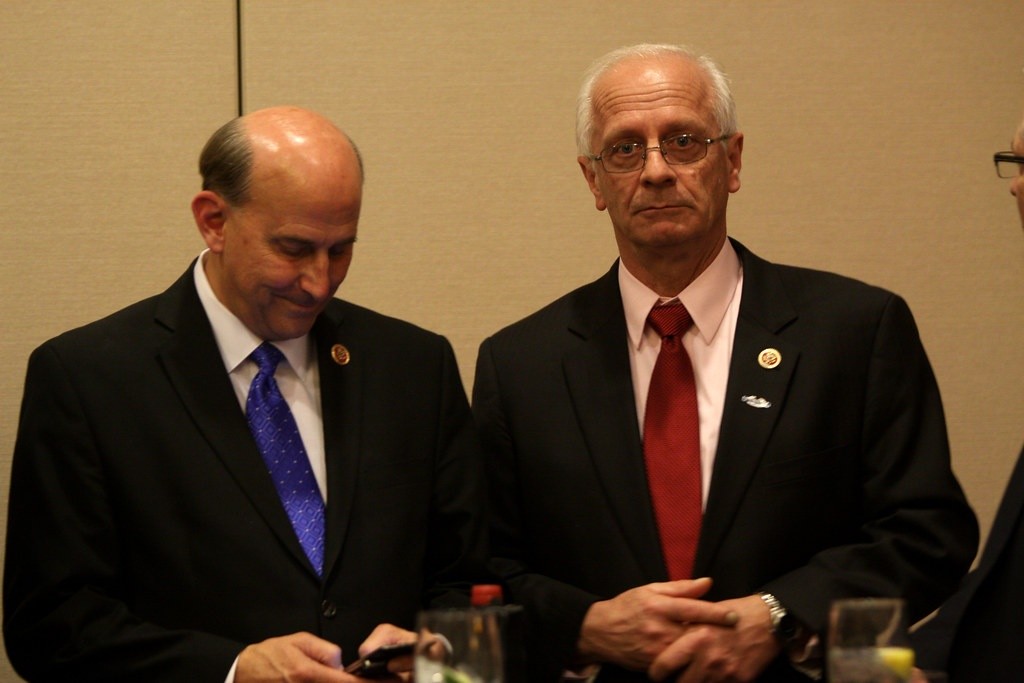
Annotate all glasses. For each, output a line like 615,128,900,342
991,148,1024,180
593,132,733,174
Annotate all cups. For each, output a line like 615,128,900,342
827,598,916,683
413,609,504,683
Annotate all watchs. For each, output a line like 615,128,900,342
762,588,818,667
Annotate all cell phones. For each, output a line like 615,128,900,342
344,642,416,679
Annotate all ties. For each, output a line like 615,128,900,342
642,302,703,580
244,343,327,579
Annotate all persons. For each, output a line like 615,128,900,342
2,104,472,683
472,43,985,682
915,108,1024,682
